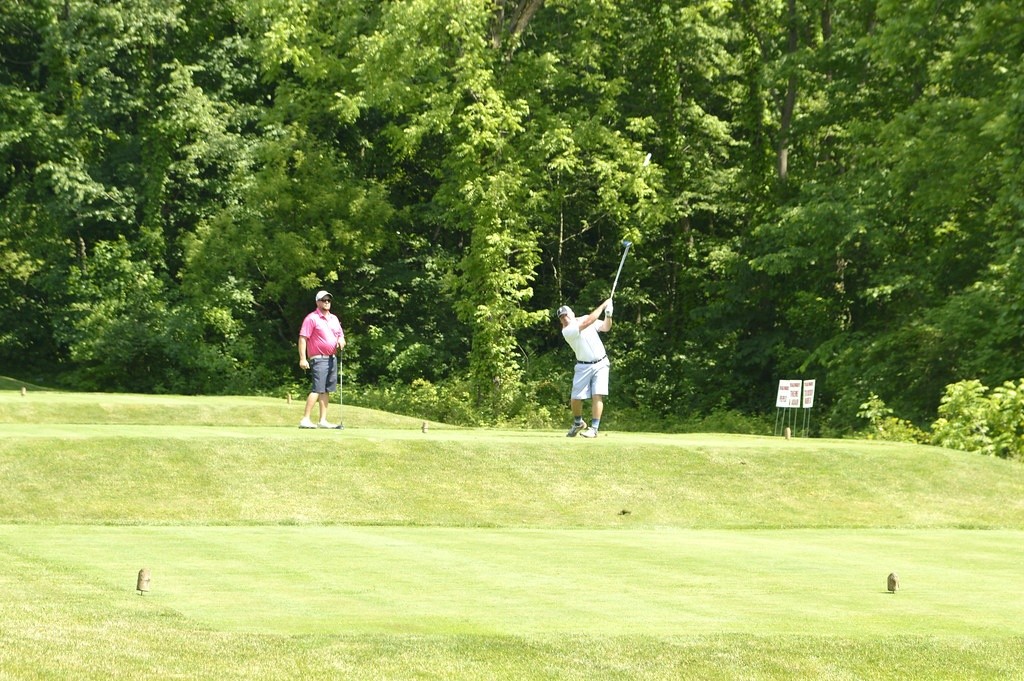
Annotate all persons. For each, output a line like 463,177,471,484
298,291,346,429
556,299,613,438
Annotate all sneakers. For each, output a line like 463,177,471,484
566,418,587,437
581,427,597,439
319,421,340,429
298,417,315,428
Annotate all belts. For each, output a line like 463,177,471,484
310,354,337,360
577,355,607,364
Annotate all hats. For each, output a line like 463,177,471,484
556,305,573,317
315,291,334,301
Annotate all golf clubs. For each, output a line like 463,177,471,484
604,241,632,315
334,350,344,429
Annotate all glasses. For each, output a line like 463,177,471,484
319,299,331,302
